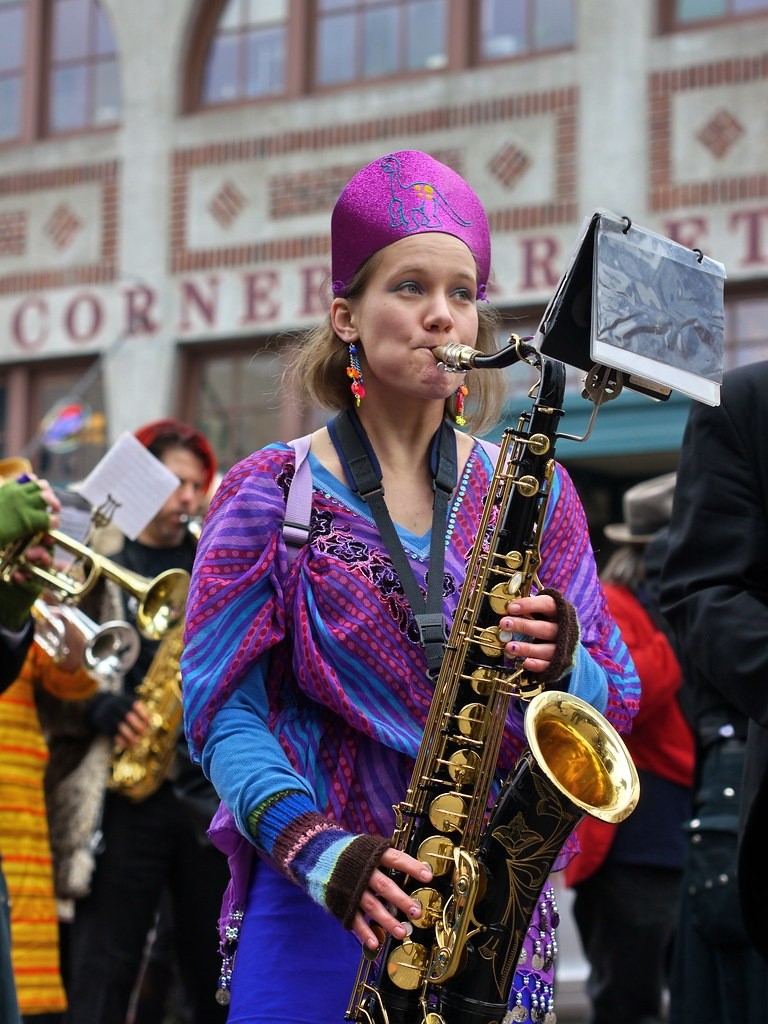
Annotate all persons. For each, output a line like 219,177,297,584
0,418,229,1024
562,472,693,1023
181,149,641,1024
663,359,768,1023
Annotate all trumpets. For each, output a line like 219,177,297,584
0,503,192,642
32,569,141,681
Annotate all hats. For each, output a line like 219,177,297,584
134,418,218,496
329,150,491,304
603,470,678,542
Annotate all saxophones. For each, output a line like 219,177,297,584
104,511,203,800
344,335,642,1024
